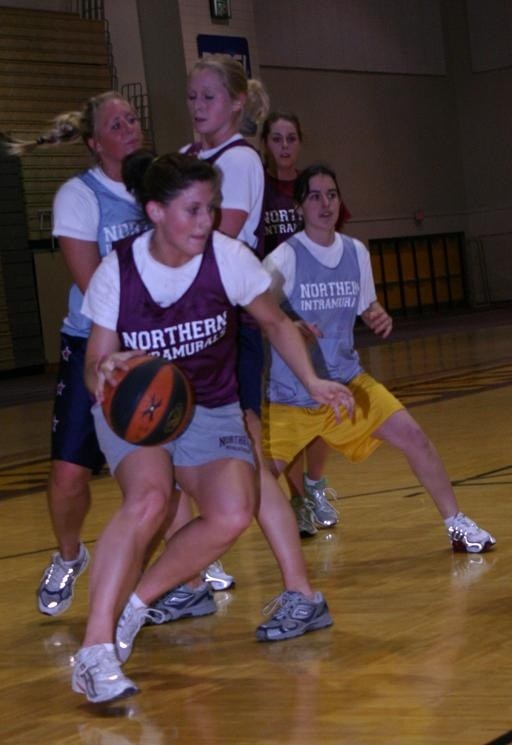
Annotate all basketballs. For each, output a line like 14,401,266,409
101,356,196,446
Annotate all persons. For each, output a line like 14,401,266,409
68,146,356,708
199,162,498,590
254,110,354,539
141,52,335,642
3,89,154,619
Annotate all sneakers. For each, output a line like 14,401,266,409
445,512,496,553
286,473,341,537
